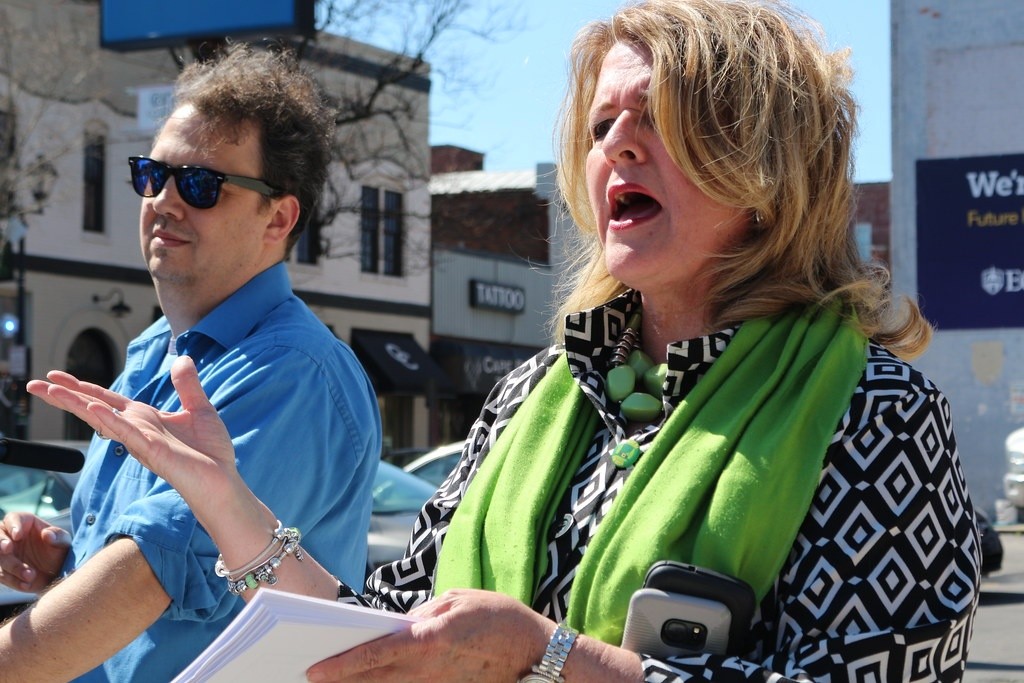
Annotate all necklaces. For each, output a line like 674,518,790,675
605,306,667,421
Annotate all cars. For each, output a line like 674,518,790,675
0,437,470,625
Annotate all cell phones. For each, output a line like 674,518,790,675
618,588,733,660
640,559,756,658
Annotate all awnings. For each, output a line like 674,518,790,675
432,339,537,395
350,331,451,396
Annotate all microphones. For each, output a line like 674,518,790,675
0,436,86,475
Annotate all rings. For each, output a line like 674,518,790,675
95,408,118,440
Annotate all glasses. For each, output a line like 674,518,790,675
128,157,289,209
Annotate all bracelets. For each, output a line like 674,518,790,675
214,519,304,596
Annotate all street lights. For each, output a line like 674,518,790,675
0,155,59,345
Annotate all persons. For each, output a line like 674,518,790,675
26,0,981,683
0,49,382,683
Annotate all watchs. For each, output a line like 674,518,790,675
516,623,580,683
